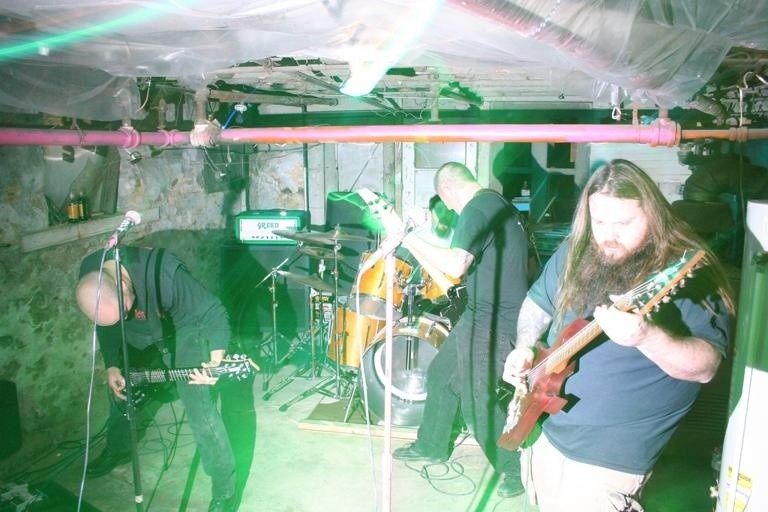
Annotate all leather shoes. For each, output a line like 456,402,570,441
84,450,136,478
500,477,527,497
209,495,236,512
393,446,448,466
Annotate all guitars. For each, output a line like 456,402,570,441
358,190,469,320
106,351,260,417
496,246,714,449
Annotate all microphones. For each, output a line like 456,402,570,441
362,207,427,269
106,209,141,252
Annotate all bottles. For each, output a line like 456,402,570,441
78,191,89,221
67,192,80,224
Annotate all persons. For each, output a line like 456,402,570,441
74,245,238,512
500,158,738,512
402,195,460,315
391,161,531,498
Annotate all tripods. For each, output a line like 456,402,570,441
277,248,371,428
263,248,352,402
254,273,310,388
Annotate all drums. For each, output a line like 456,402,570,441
349,248,412,321
357,312,455,428
328,296,404,369
421,267,462,306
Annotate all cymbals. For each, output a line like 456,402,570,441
307,230,375,245
275,269,335,295
272,228,336,247
299,247,346,262
